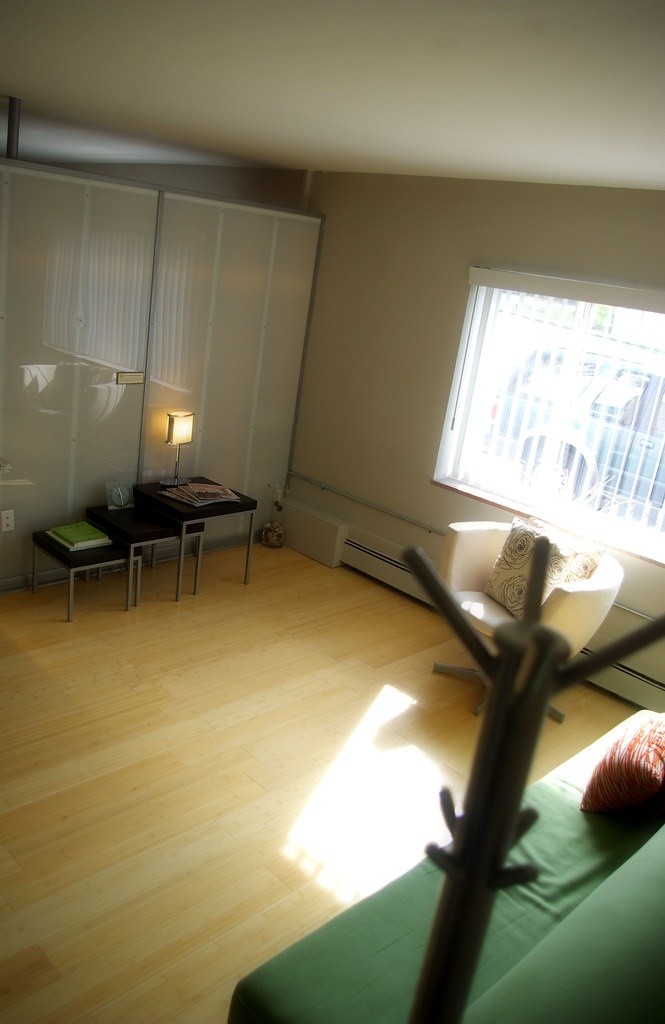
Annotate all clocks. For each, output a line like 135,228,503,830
105,481,135,509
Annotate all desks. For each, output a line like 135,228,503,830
85,505,204,611
132,476,257,601
32,523,144,621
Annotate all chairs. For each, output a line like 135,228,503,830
431,516,623,723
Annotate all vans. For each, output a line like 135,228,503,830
485,349,665,532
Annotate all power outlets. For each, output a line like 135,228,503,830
2,508,16,532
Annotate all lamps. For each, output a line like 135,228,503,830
160,412,196,486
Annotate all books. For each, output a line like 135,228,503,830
45,521,112,551
159,483,241,506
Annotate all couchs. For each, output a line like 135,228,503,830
227,708,665,1023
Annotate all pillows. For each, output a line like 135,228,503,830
579,712,665,813
486,518,601,618
